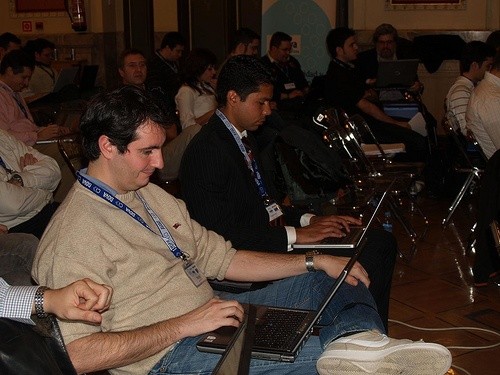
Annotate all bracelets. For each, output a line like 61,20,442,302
34,286,50,318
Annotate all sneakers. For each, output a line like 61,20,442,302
316,329,452,375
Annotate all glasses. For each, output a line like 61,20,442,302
377,39,392,45
42,54,52,57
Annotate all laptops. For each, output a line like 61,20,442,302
35,134,75,144
195,238,368,363
291,177,398,249
53,65,100,102
211,307,257,375
376,58,420,88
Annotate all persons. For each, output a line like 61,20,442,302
322,26,432,172
31,84,451,375
445,40,489,137
351,23,424,96
0,277,113,325
465,30,500,160
0,129,64,240
0,32,99,148
113,30,311,181
177,54,397,337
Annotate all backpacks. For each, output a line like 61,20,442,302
273,124,353,195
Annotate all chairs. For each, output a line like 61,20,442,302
308,84,500,275
57,133,88,176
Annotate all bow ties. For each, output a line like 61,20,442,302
241,136,252,149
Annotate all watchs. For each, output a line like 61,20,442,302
305,250,323,273
11,174,24,186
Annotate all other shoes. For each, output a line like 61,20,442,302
410,188,434,201
